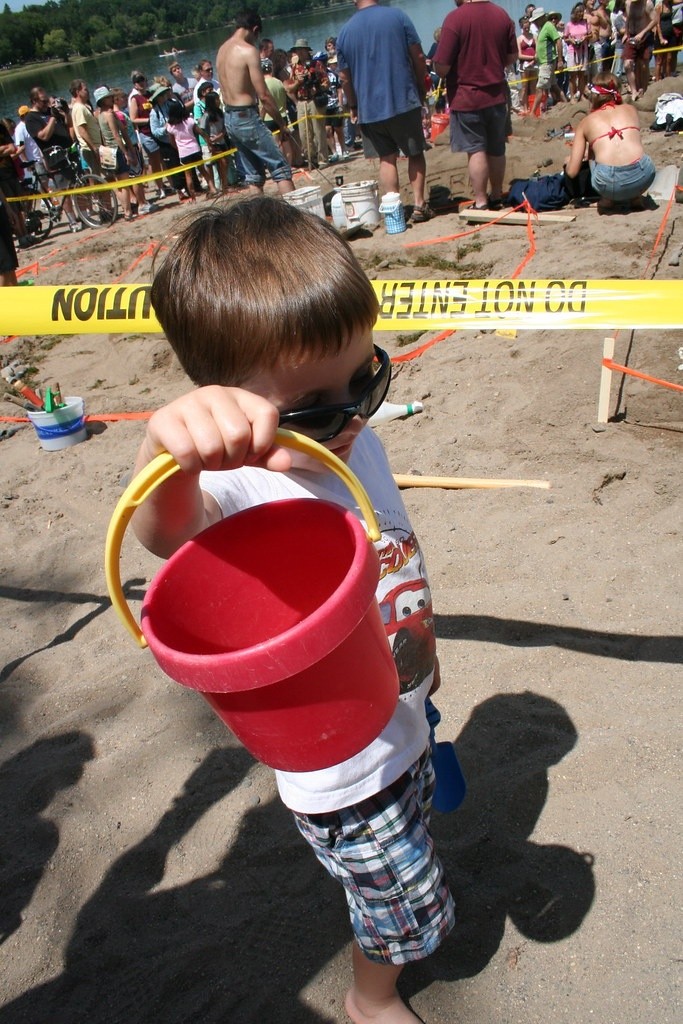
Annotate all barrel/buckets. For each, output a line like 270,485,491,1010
26,397,87,450
430,114,450,143
282,180,379,231
105,426,400,774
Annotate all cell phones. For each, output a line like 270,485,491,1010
20,141,24,146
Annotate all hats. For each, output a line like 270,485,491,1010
18,105,30,116
547,11,562,20
146,83,171,102
528,7,548,22
93,87,113,103
290,39,313,51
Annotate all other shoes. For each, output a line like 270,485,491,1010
19,236,42,247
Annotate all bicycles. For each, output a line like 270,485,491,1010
12,140,119,242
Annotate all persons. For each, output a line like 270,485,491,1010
433,0,518,211
564,71,656,207
129,190,456,1024
423,0,683,148
0,38,363,286
215,12,296,197
336,0,437,222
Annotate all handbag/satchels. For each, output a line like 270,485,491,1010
588,45,595,61
315,93,328,107
99,145,118,171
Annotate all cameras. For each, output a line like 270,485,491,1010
574,40,581,48
629,39,639,48
55,100,62,108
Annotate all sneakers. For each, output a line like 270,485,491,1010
138,201,158,215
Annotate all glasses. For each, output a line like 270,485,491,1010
278,343,391,443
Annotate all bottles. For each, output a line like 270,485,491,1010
379,192,406,234
365,400,423,426
70,141,77,153
330,187,347,228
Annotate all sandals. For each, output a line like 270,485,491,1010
411,204,436,221
467,194,507,210
